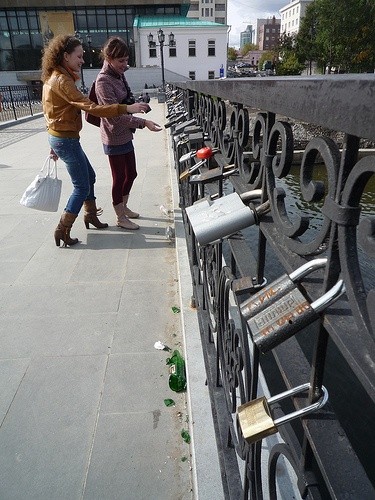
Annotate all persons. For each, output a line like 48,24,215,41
94,36,162,230
40,33,152,246
138,92,150,103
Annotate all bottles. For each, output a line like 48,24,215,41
169,350,185,392
165,226,173,239
160,203,168,215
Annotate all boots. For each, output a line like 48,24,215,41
123,195,139,218
83,199,108,229
113,201,140,229
54,210,79,246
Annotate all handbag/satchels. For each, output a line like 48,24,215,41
20,155,62,212
85,81,101,127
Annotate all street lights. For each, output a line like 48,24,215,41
73,29,93,94
147,28,176,88
